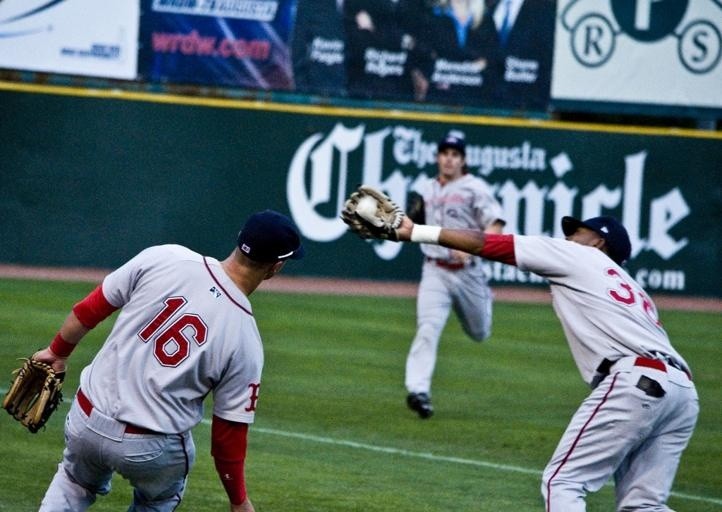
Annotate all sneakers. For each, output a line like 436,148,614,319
405,392,435,419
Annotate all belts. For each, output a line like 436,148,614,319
426,255,478,269
76,389,159,436
633,354,668,371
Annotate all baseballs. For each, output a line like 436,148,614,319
356,195,378,216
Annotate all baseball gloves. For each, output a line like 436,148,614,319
338,184,404,241
2,354,66,433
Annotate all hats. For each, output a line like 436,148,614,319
561,214,632,263
437,136,468,154
236,209,305,262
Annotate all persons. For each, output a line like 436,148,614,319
401,128,506,419
34,209,303,512
339,185,702,512
